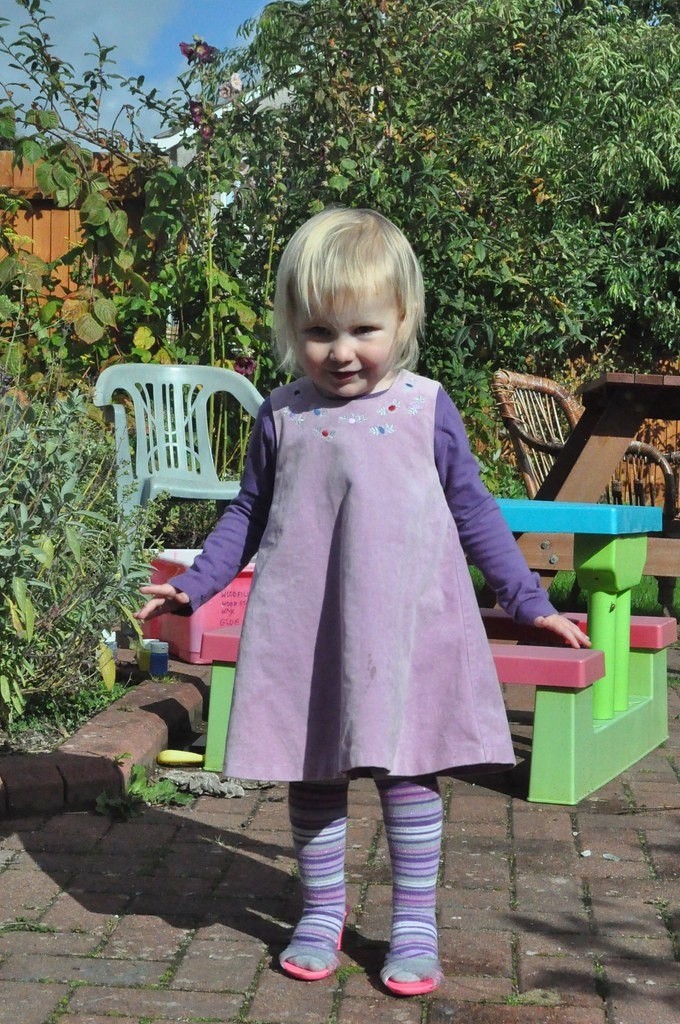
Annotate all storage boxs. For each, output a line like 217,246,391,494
139,542,258,664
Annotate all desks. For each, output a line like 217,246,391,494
203,497,669,806
482,373,680,613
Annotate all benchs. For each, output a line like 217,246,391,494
200,612,677,690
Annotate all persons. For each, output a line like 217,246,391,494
133,209,593,995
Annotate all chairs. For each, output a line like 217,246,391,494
94,363,265,555
490,370,673,540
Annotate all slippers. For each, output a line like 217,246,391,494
379,953,445,995
278,902,350,980
